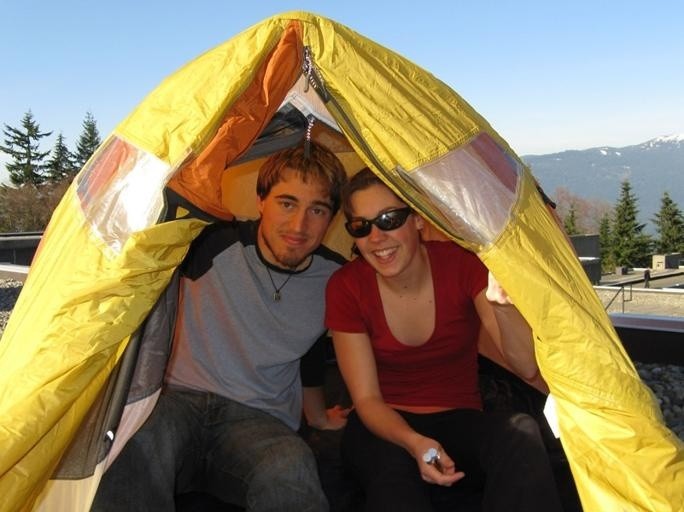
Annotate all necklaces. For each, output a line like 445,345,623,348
263,254,298,303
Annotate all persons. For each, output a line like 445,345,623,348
89,139,354,512
324,166,564,511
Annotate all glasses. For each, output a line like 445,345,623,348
345,208,411,237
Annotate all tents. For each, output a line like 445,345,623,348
0,10,683,512
